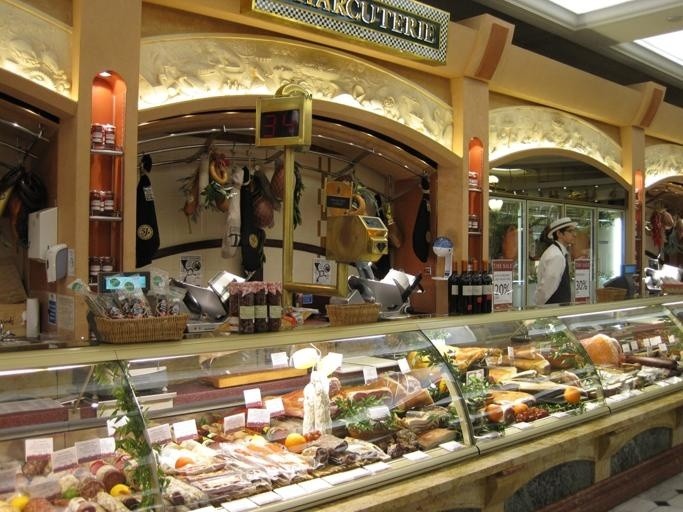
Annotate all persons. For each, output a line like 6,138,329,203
533,217,577,305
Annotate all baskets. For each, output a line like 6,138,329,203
325,302,381,326
662,283,682,293
94,311,189,344
595,286,626,302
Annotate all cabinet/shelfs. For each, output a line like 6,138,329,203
0,292,683,511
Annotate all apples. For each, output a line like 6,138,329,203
486,405,504,422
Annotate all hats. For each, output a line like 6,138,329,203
546,217,576,239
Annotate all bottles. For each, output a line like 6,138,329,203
227,281,284,335
447,257,494,315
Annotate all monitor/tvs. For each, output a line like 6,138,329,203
603,275,633,297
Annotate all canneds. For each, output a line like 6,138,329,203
92,124,115,151
89,256,113,283
89,189,115,216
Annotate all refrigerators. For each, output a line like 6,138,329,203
488,190,630,310
